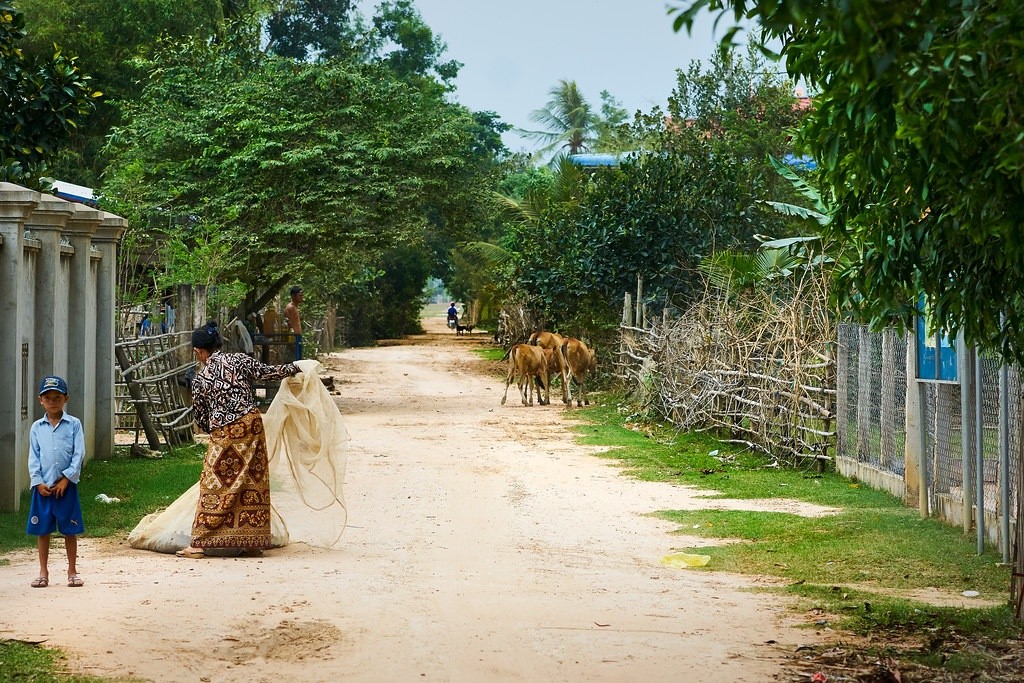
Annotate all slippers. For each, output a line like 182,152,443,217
31,577,48,588
247,549,263,556
176,548,204,558
68,577,83,587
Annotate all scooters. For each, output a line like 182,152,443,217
445,310,460,330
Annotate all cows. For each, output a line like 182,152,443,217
499,330,598,408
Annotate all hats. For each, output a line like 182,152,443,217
38,376,67,395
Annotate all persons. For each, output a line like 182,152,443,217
25,376,84,587
176,320,300,558
447,302,457,324
284,285,303,360
